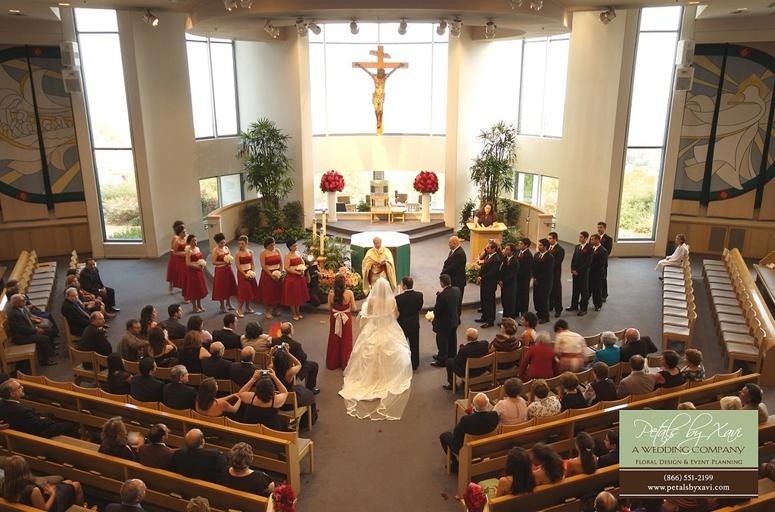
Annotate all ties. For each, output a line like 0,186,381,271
551,247,553,251
580,246,583,251
540,254,543,259
593,248,596,252
448,250,452,257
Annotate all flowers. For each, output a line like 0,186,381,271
413,170,439,195
319,168,345,193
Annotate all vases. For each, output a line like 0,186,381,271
327,191,338,223
421,193,430,224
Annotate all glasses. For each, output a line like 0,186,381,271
16,384,22,393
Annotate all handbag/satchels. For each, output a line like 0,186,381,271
127,431,146,449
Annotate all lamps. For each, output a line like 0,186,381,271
263,15,321,39
348,15,360,34
143,7,159,28
396,15,408,35
437,14,496,39
600,6,616,25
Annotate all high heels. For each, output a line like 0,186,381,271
183,297,303,321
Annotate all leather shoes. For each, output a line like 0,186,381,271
474,294,608,328
91,505,97,512
444,384,458,391
103,324,111,338
432,361,443,367
313,388,321,395
104,311,117,320
111,307,121,312
84,502,88,508
433,354,439,360
49,341,61,357
40,361,57,367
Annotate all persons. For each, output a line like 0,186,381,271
235,235,257,317
394,277,424,369
183,233,209,313
0,255,321,512
430,273,461,367
655,233,690,281
475,222,613,329
282,239,310,321
356,277,402,370
474,202,496,227
355,62,405,130
258,238,281,318
435,307,775,511
211,233,236,311
441,235,466,326
166,221,186,295
325,275,357,370
362,237,396,295
172,225,193,304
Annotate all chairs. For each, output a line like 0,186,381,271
368,191,408,224
337,196,356,213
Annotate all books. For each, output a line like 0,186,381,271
375,258,386,266
